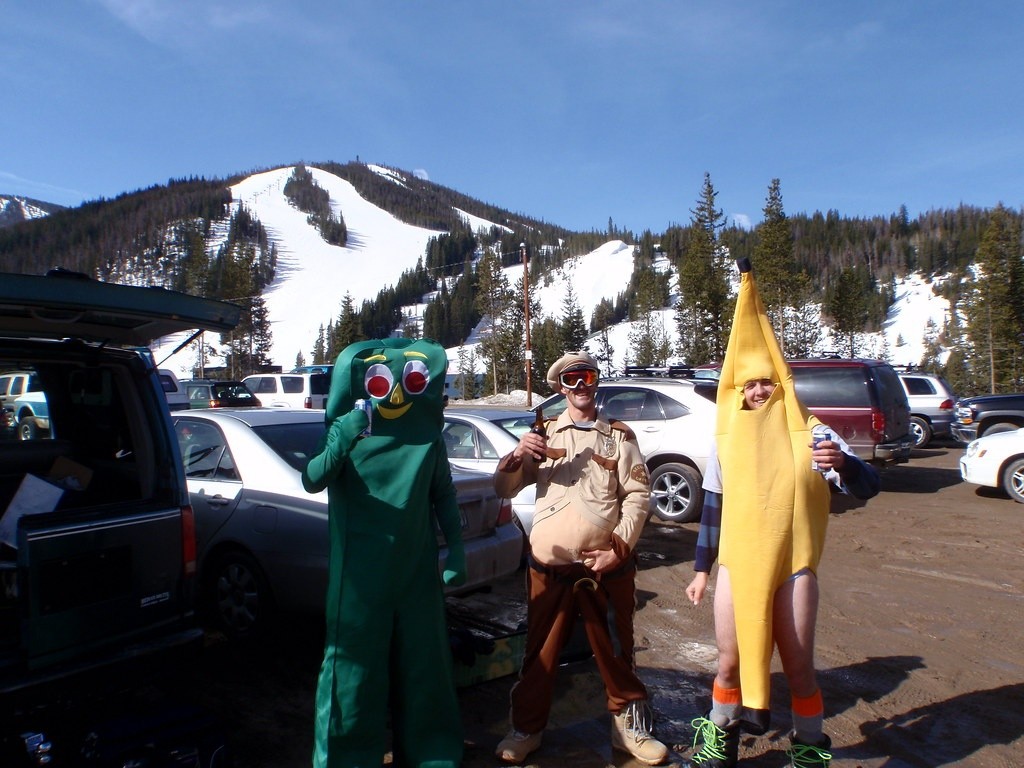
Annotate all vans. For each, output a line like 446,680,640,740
0,268,253,692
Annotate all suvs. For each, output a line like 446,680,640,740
891,364,958,449
177,378,262,409
529,378,720,524
0,371,39,428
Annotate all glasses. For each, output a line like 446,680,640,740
558,367,599,390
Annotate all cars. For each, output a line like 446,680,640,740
442,410,548,539
950,392,1024,443
959,428,1024,504
166,403,524,646
157,369,192,412
14,392,50,440
218,373,330,410
694,360,911,462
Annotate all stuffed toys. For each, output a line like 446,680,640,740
300,338,478,768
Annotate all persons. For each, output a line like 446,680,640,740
493,350,669,766
678,257,881,768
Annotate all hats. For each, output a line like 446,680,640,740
546,350,598,392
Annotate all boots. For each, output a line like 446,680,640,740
788,730,832,768
676,713,740,768
610,700,669,765
495,728,544,765
